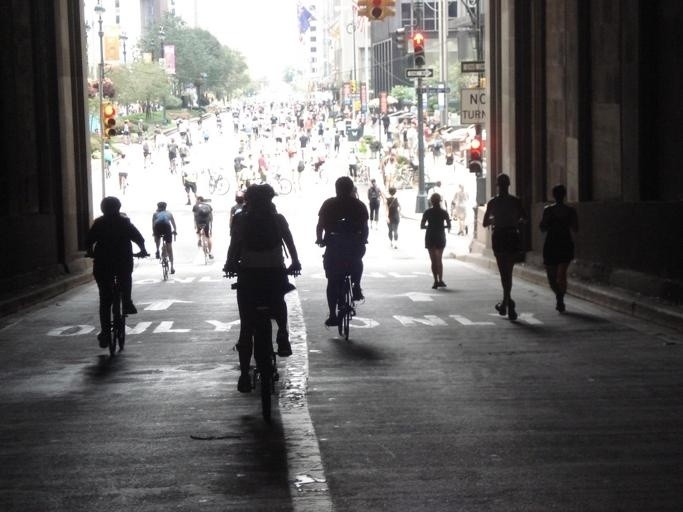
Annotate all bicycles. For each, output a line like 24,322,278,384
111,139,381,205
388,167,428,190
149,230,177,281
196,223,210,266
82,251,151,355
314,238,358,342
217,265,304,420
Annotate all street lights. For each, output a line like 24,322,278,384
90,0,108,205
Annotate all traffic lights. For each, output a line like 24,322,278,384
392,27,408,53
100,100,116,138
383,1,396,18
367,1,385,22
412,30,427,67
357,1,370,23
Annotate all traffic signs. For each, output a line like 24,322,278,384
415,87,452,94
402,67,435,79
459,61,485,72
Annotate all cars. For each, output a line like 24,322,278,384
384,108,488,180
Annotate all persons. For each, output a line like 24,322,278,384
313,175,370,330
82,194,149,350
221,184,301,394
480,171,531,321
93,95,469,289
537,180,584,315
